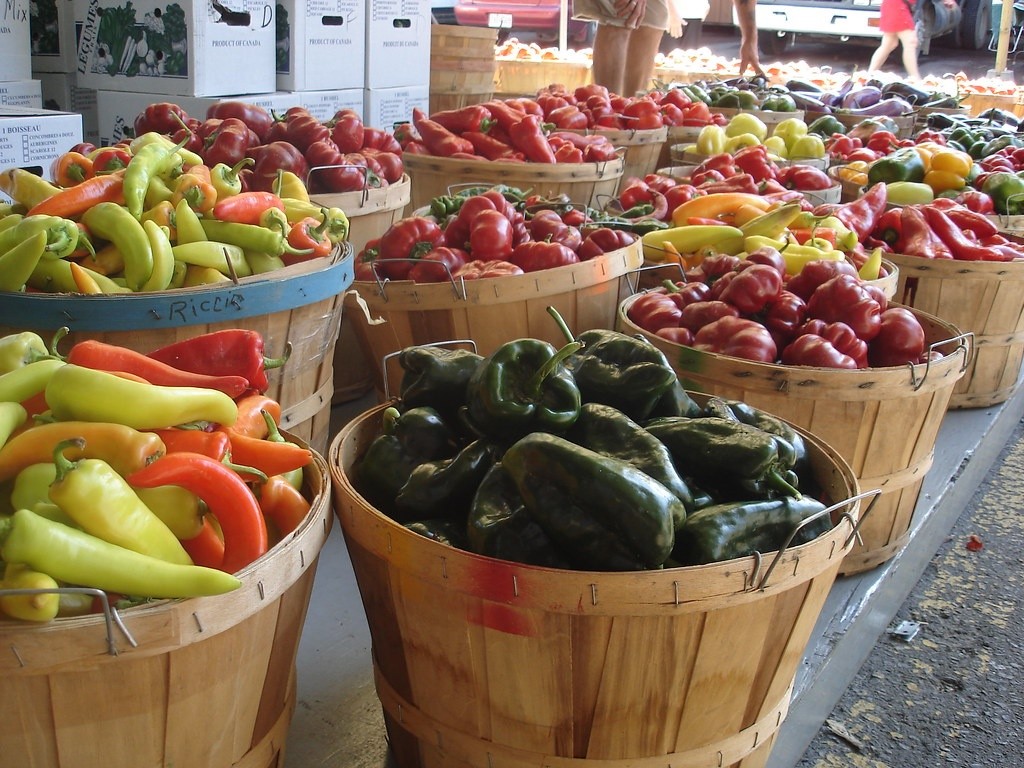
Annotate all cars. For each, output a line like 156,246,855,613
452,0,597,48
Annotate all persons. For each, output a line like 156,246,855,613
868,0,921,79
571,0,771,98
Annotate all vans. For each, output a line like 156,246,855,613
730,0,992,57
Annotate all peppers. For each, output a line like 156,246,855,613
0,83,1024,621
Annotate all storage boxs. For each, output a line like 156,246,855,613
0,0,433,208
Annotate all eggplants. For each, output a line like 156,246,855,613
658,73,1024,135
